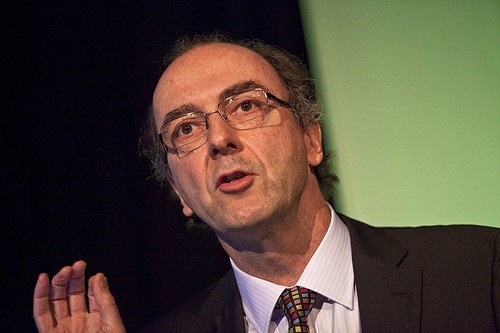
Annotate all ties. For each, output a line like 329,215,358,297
276,286,319,333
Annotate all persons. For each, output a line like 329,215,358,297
31,34,499,333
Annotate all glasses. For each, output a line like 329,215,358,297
159,88,295,165
